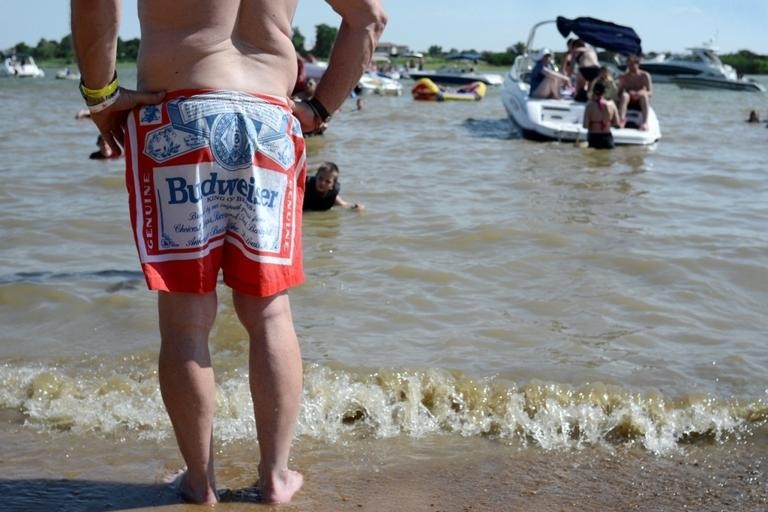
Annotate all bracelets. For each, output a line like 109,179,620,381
309,98,331,124
77,71,120,114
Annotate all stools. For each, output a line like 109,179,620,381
620,109,641,128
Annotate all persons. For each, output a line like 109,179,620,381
619,54,653,130
356,99,365,109
68,1,390,505
582,81,622,149
299,160,367,213
372,58,425,72
746,110,759,123
530,37,618,100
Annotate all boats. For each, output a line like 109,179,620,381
672,71,766,93
53,67,80,81
411,78,486,102
500,15,662,147
408,55,506,86
365,57,400,81
355,72,400,98
4,54,44,79
639,29,739,82
391,52,436,77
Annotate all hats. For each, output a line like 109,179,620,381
533,48,555,65
